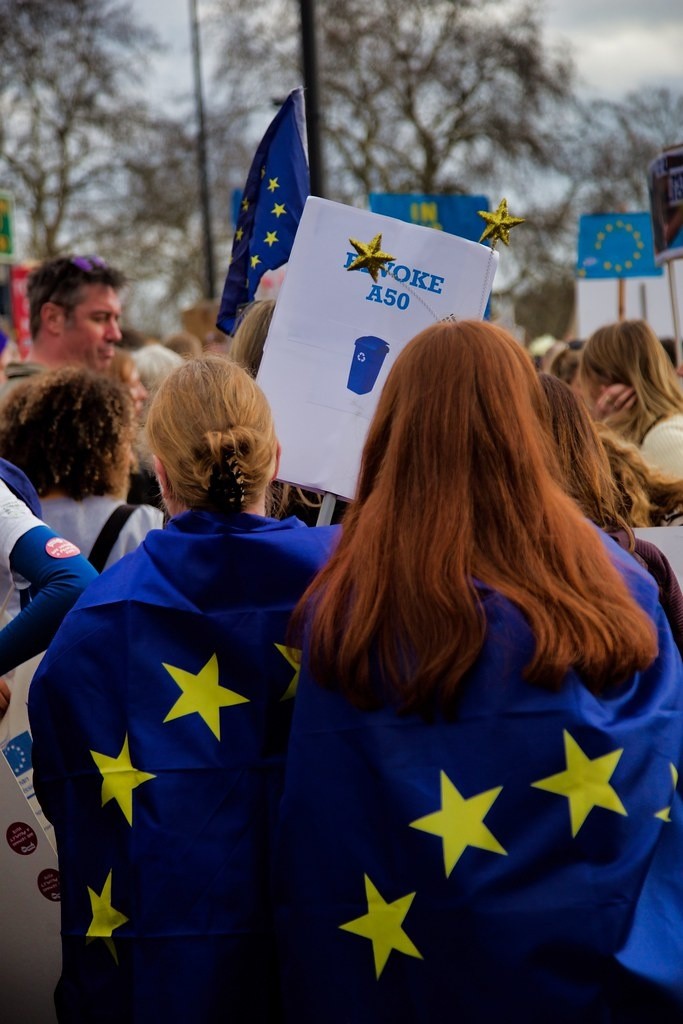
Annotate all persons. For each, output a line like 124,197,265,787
0,249,683,1024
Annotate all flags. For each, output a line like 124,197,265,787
378,191,500,321
212,87,309,338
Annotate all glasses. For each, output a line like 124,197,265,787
47,256,110,309
